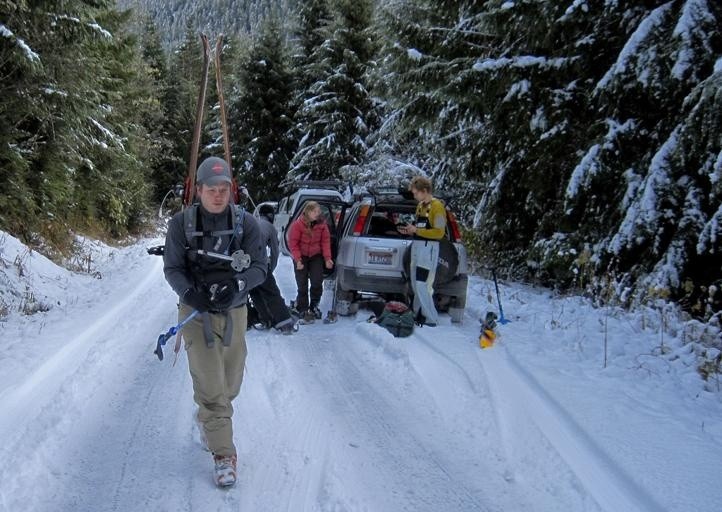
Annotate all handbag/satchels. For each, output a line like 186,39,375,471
380,308,414,336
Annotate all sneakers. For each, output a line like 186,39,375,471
301,307,321,319
213,452,236,487
282,324,292,334
195,412,214,452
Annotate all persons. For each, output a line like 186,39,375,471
384,212,400,225
396,175,447,327
247,204,292,333
161,156,280,488
286,198,335,320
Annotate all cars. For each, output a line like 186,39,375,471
332,187,467,323
272,179,344,257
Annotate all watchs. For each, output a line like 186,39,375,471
238,279,245,292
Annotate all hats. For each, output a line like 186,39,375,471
197,157,233,187
259,204,274,221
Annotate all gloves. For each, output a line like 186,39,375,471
180,288,210,312
211,280,239,313
